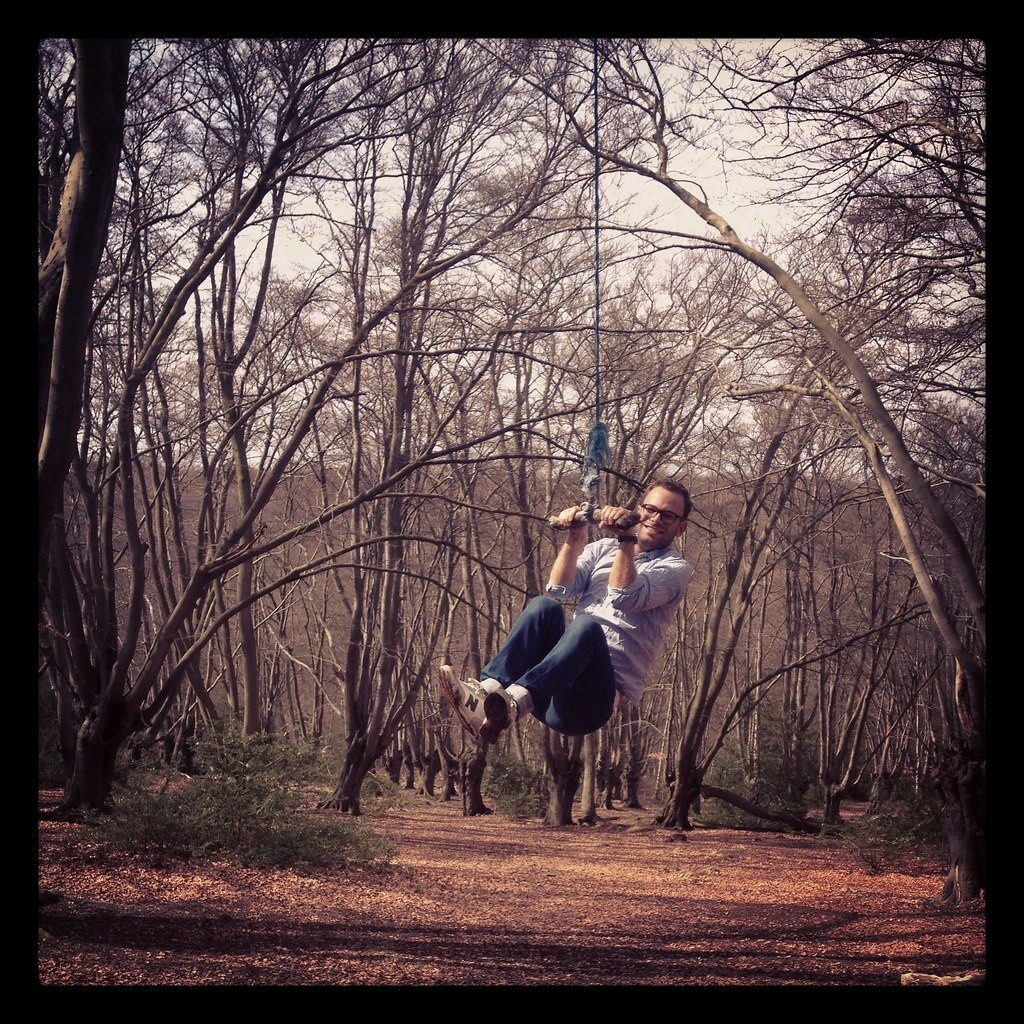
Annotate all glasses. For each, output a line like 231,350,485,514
641,503,683,526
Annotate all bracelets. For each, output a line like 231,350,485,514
617,535,639,544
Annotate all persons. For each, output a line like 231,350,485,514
436,476,697,744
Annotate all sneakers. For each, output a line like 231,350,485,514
478,688,517,745
438,664,489,736
